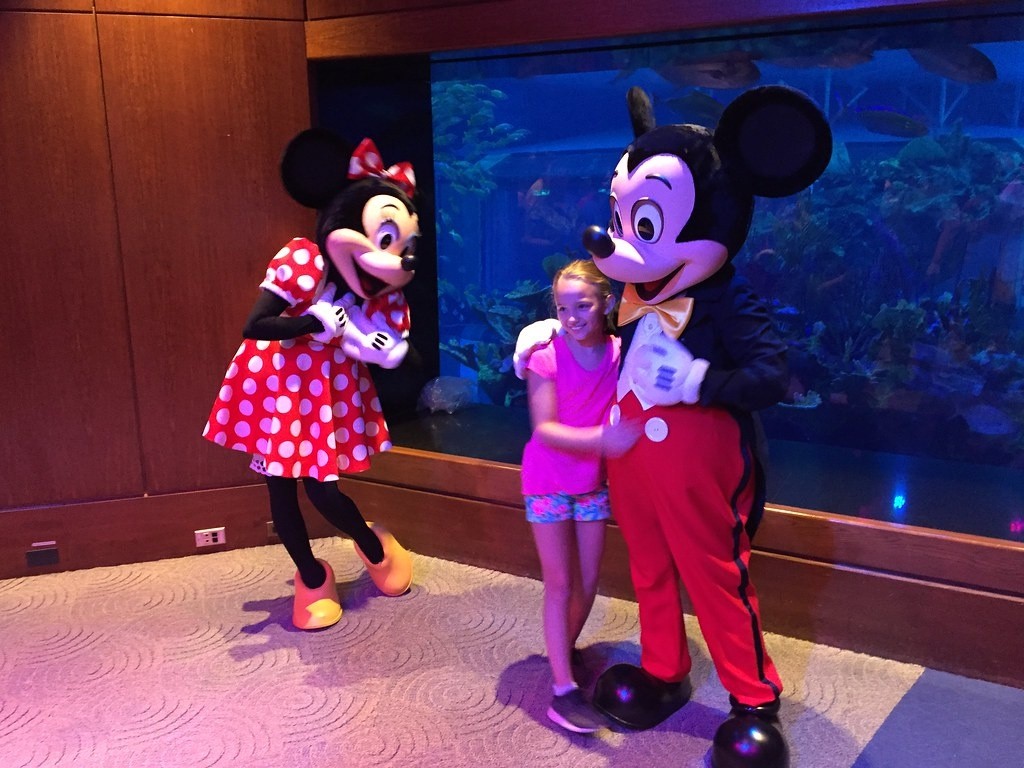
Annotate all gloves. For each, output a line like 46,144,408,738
341,305,409,369
304,282,360,344
513,319,568,380
633,335,711,405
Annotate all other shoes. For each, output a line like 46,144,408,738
592,664,692,730
711,693,789,768
292,558,342,629
353,521,413,596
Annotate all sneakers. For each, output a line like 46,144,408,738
569,648,588,690
547,692,599,732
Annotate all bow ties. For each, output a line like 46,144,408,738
618,282,694,341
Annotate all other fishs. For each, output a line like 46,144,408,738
650,34,997,138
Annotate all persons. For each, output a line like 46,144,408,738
202,127,435,630
924,169,1024,361
520,258,642,735
513,84,833,767
